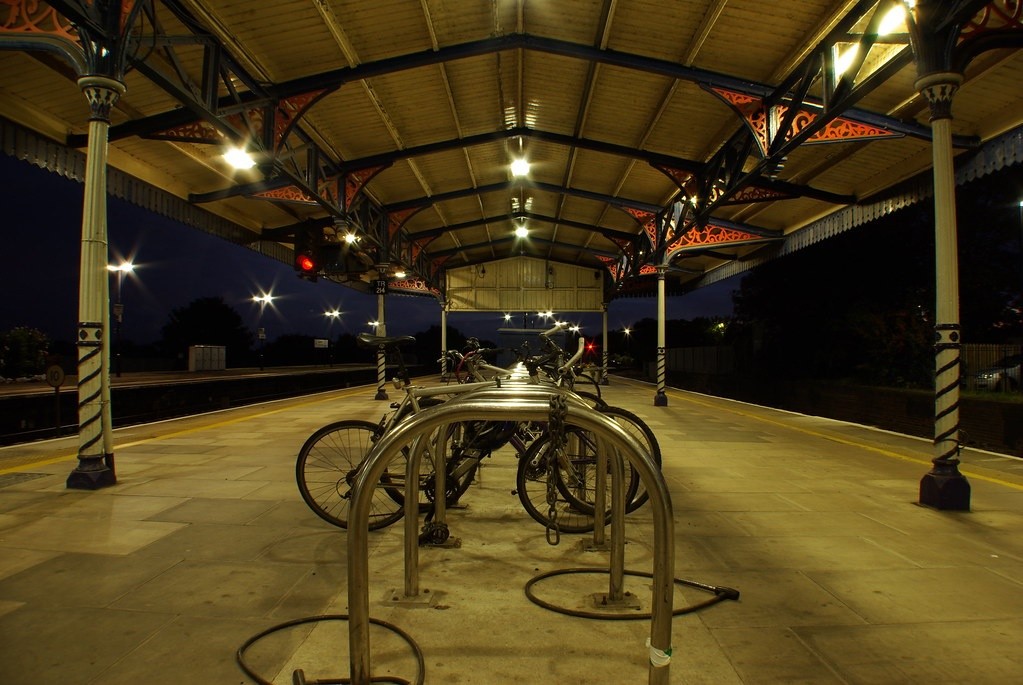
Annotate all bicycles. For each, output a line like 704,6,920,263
294,323,663,536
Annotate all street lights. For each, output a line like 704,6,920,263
324,311,339,367
107,261,133,378
252,294,272,370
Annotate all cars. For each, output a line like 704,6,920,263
974,354,1023,393
